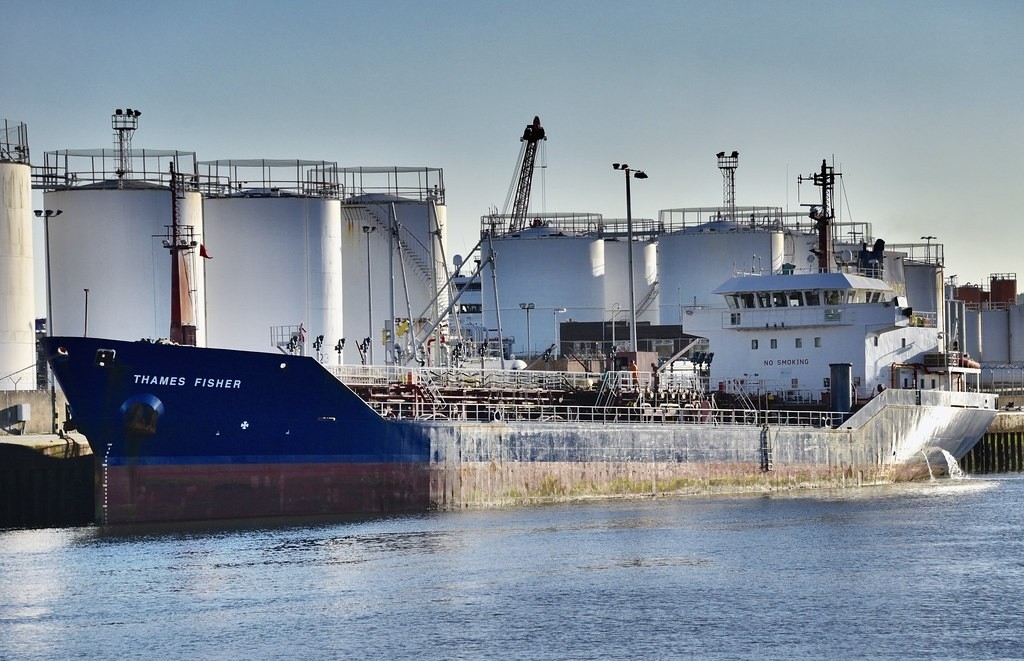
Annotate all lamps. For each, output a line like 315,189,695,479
685,310,693,316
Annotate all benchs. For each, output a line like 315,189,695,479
643,408,693,424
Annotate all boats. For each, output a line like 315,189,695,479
36,143,1000,529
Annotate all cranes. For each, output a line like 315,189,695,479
494,114,549,235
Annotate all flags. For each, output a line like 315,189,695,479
200,245,213,259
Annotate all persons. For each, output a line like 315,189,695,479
701,396,711,424
858,239,885,302
629,360,640,388
650,363,660,392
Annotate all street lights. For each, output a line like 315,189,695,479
84,288,89,338
33,209,64,436
362,225,376,366
613,162,649,353
520,302,536,358
554,307,566,360
